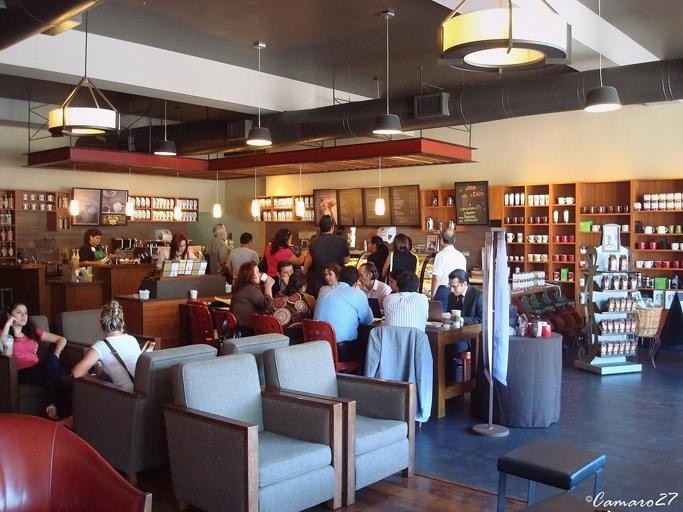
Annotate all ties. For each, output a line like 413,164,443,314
457,295,465,316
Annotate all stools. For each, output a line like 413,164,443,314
512,486,609,511
493,438,609,511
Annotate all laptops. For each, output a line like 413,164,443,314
368,298,385,318
428,300,448,321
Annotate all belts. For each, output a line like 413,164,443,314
338,340,360,344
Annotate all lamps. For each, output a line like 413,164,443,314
59,157,394,219
153,97,179,156
370,8,406,138
433,1,574,75
245,41,273,148
583,1,624,115
47,6,120,135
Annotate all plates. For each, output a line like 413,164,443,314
425,321,442,328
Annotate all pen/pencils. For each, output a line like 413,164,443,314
31,256,38,265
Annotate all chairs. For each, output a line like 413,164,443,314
263,338,419,510
161,351,346,512
70,345,215,488
0,413,154,512
0,342,84,418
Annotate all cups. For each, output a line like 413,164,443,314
441,312,450,329
634,220,641,233
22,193,54,211
504,192,574,224
127,196,197,222
515,267,519,274
621,224,629,231
432,197,437,206
518,271,545,282
579,248,587,305
581,206,629,214
451,310,461,328
542,324,551,336
642,224,681,234
446,196,453,206
634,238,683,268
553,272,560,281
259,197,314,222
260,273,274,285
1,335,13,356
568,271,574,281
506,232,575,243
591,224,600,232
519,280,544,291
527,323,532,334
86,273,92,282
189,289,197,300
506,254,575,261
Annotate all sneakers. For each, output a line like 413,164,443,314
45,404,58,422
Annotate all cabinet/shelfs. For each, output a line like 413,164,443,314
0,176,682,377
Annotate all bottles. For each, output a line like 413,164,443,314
667,275,679,289
532,311,542,336
362,239,367,251
70,248,79,270
635,271,654,289
58,197,69,229
0,193,14,258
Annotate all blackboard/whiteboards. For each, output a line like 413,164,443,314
455,181,489,225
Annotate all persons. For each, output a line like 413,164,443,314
78,229,117,275
71,300,143,390
155,231,203,279
0,300,67,421
225,215,484,402
207,223,231,275
